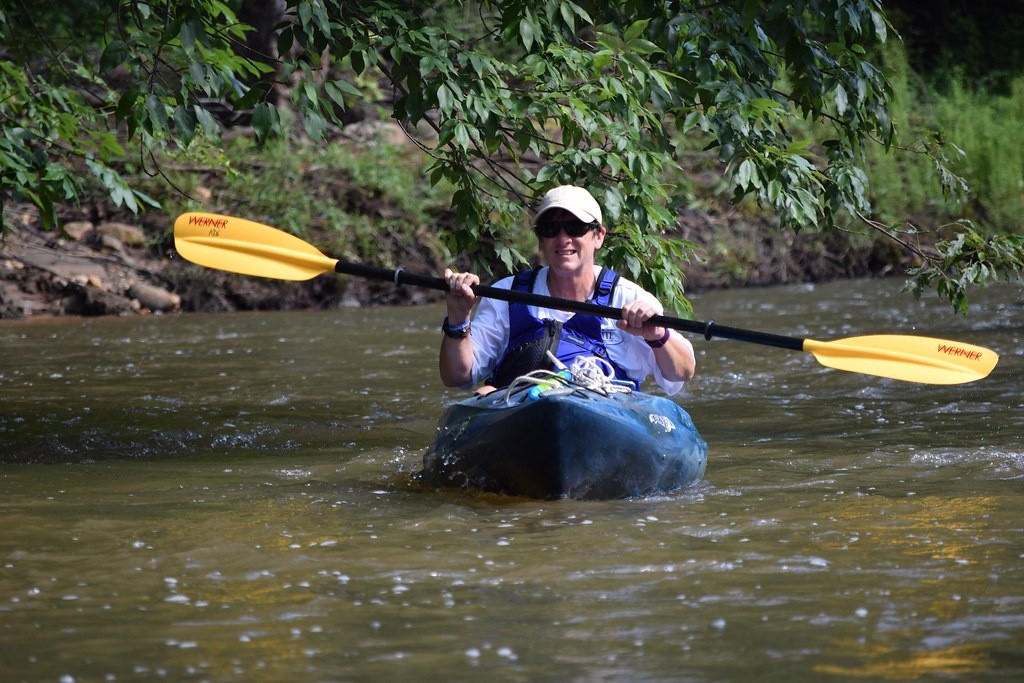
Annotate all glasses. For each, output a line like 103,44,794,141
535,221,598,238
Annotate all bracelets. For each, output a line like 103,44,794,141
441,316,472,340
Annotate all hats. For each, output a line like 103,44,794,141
536,185,603,228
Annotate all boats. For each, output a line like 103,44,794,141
420,374,709,502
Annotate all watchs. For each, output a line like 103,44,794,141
644,327,670,348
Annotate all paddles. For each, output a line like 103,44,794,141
167,204,1001,390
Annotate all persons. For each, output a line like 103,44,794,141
439,185,696,396
